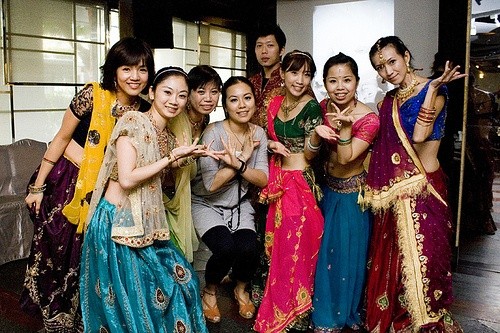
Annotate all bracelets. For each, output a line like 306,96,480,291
29,183,47,193
42,157,57,166
237,154,246,165
338,137,353,146
267,139,274,154
163,154,172,167
307,136,322,153
236,160,247,175
171,151,177,161
177,156,197,168
416,105,437,128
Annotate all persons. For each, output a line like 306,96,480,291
358,36,468,333
251,50,341,333
248,24,317,309
460,59,496,239
427,48,464,226
310,52,380,333
191,75,269,323
20,35,155,333
80,66,226,333
161,64,223,270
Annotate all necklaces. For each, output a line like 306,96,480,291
280,94,303,118
396,73,420,101
228,122,251,151
188,112,204,132
330,97,357,129
147,110,168,157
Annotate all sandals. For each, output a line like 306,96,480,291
201,289,221,322
234,287,255,318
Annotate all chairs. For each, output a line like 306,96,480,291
0,139,48,266
